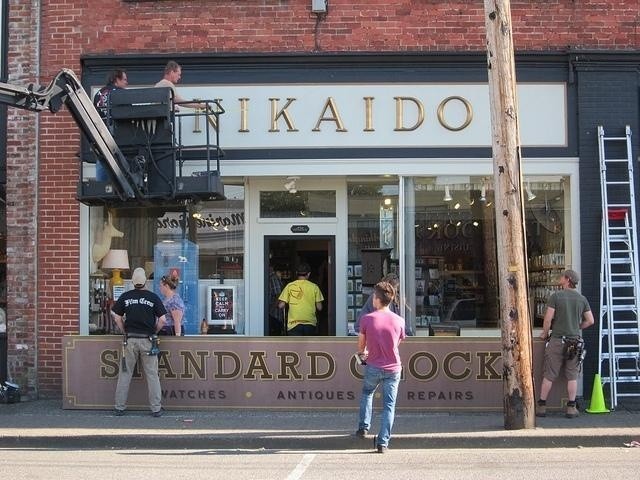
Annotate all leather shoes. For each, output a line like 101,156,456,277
152,408,164,417
566,406,579,418
114,408,126,416
356,429,367,440
377,445,387,453
535,404,546,417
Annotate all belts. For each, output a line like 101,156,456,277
128,336,148,338
559,336,580,340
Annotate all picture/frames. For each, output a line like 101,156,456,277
205,284,240,327
346,262,440,335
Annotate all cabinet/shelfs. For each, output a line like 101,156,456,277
445,271,477,329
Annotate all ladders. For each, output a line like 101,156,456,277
597,124,640,411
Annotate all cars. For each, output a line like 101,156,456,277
415,270,476,332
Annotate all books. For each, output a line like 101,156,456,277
355,352,369,365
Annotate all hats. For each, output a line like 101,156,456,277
132,267,146,288
295,262,312,276
565,270,579,285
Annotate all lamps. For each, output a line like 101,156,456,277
382,193,393,207
101,247,131,297
450,199,462,210
426,212,479,230
478,182,488,202
525,178,537,202
442,181,453,202
283,176,302,195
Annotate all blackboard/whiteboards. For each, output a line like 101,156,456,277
206,285,237,326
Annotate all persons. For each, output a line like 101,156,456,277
93,67,130,182
152,59,211,112
354,272,417,335
278,262,324,334
267,260,286,334
110,265,167,416
353,279,406,452
534,269,595,418
156,273,187,336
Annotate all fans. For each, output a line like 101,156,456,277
529,182,562,233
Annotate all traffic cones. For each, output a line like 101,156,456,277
585,373,610,414
